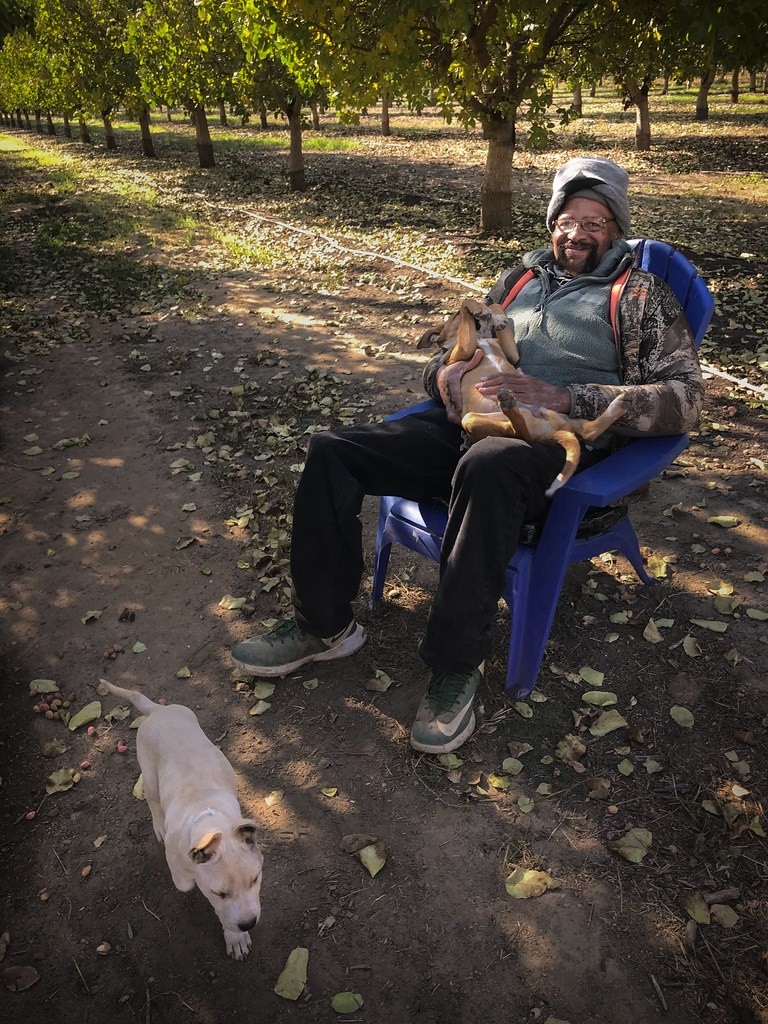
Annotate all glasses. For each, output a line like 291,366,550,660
552,216,616,232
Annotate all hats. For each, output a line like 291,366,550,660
546,156,632,236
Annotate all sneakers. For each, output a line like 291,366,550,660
231,618,368,677
410,658,485,754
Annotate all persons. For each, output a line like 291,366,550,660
232,159,705,753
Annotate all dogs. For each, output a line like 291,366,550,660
417,299,637,497
100,679,263,961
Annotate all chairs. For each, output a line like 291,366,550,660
371,239,717,700
